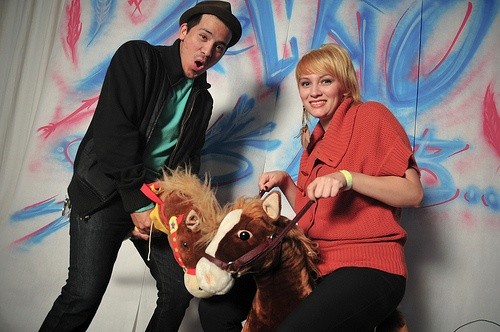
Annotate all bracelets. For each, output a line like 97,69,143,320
338,169,352,191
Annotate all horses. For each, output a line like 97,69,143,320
196,190,407,332
151,159,225,299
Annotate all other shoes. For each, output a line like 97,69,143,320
392,319,409,332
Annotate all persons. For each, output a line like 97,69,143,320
258,43,424,332
38,0,243,332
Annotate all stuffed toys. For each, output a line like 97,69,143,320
196,189,408,332
135,160,223,299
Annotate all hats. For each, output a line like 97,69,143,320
179,0,242,47
